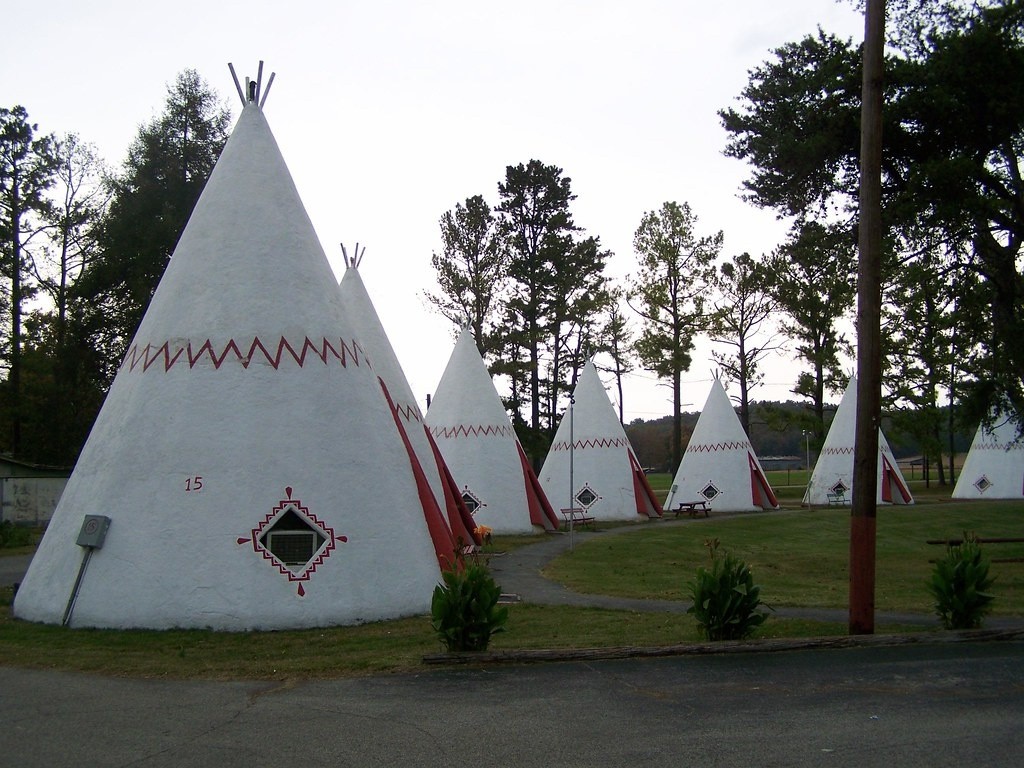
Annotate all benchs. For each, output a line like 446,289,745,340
826,494,850,508
671,502,698,517
453,535,478,569
688,501,711,518
561,508,595,528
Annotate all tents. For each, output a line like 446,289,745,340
426,311,560,536
663,368,780,512
952,379,1024,500
13,57,461,631
801,367,915,506
337,241,483,553
538,350,663,521
